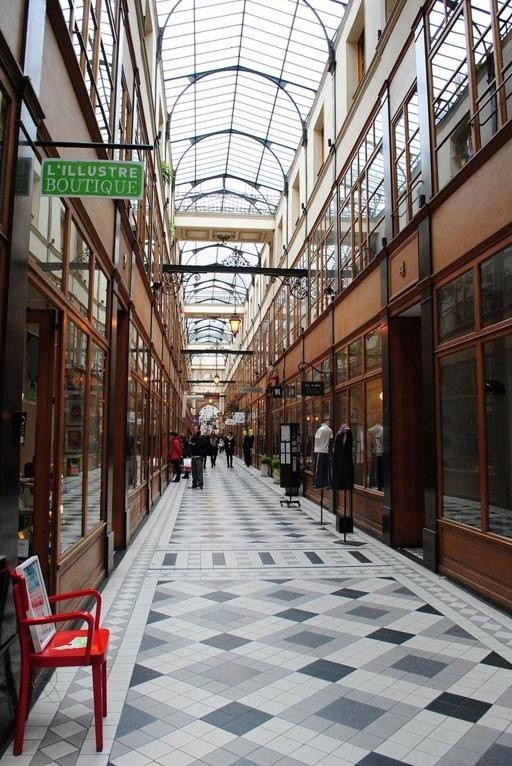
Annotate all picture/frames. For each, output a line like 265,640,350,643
62,427,87,455
63,395,88,427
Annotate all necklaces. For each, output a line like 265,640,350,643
168,424,224,492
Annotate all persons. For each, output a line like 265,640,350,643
242,424,254,467
333,422,354,492
367,423,383,490
224,431,235,469
311,420,334,491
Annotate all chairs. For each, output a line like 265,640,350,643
4,547,110,757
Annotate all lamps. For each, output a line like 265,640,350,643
228,254,243,336
213,340,221,388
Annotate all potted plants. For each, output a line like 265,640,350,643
270,459,282,484
258,454,270,478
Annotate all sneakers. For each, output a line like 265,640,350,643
171,472,181,482
192,480,203,490
181,471,189,479
227,464,233,468
204,463,206,469
211,464,216,468
